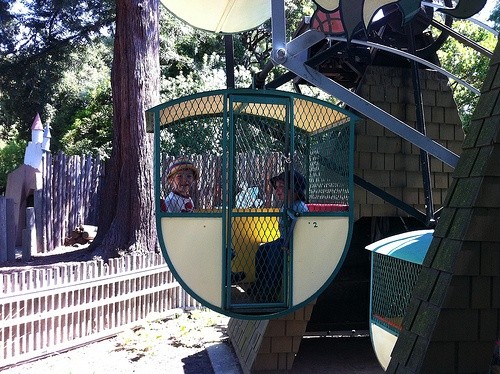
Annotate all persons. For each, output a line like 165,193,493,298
255,170,310,302
163,158,246,305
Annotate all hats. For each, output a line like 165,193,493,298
166,157,199,179
271,171,307,200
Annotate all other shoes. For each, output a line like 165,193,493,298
226,272,246,285
